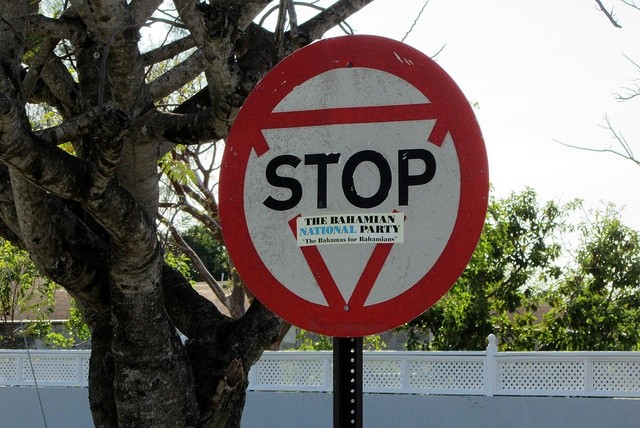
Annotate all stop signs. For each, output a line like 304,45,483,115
218,33,490,338
